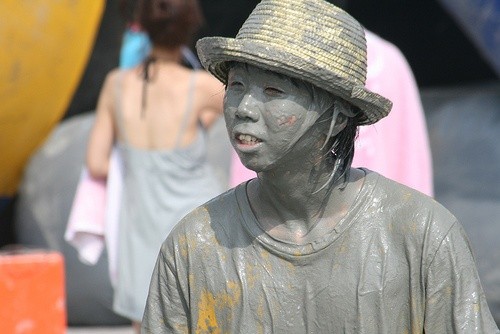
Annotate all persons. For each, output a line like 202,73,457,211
119,6,151,69
229,26,433,199
88,0,231,334
141,0,500,334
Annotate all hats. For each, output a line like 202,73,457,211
195,0,393,127
